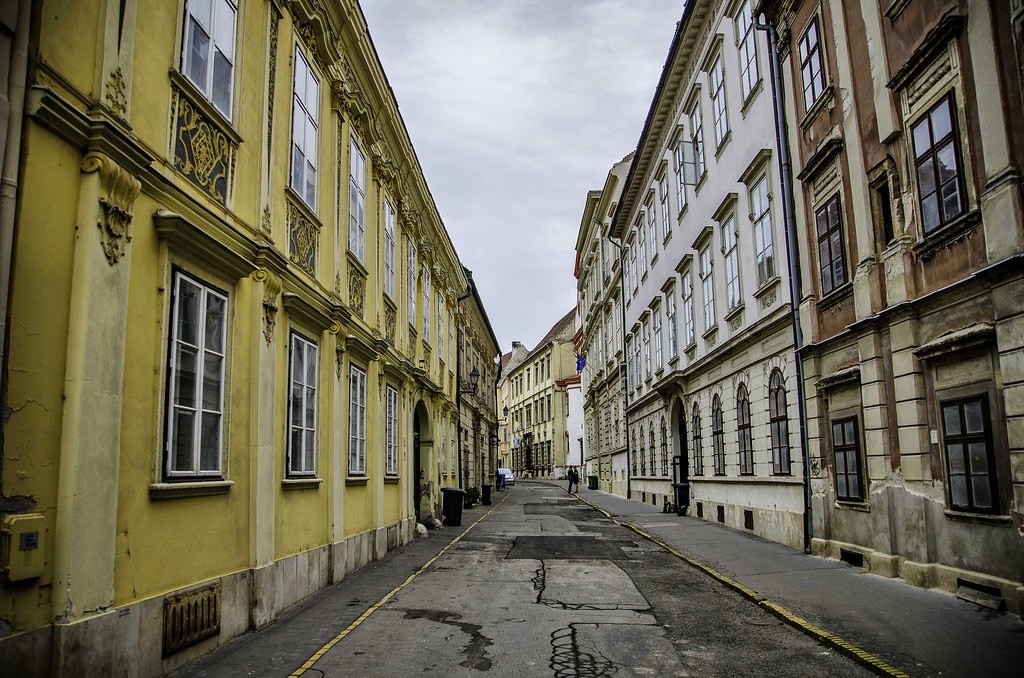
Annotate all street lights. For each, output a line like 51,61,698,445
578,438,583,479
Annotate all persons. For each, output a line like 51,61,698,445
573,466,579,493
567,465,573,494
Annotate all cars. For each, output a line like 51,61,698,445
498,468,515,485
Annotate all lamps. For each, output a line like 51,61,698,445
458,366,480,398
495,405,509,422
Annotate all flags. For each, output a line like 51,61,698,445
513,438,518,446
576,354,585,370
516,422,520,430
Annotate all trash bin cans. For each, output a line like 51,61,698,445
440,488,467,527
587,476,598,489
481,484,494,505
